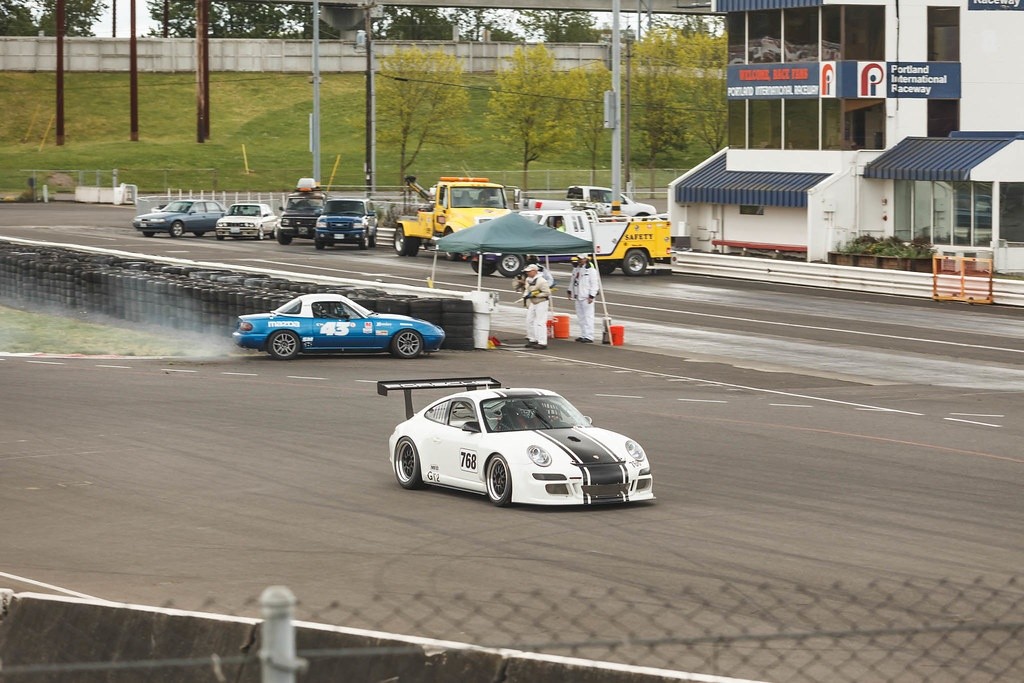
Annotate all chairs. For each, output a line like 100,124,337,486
458,191,473,207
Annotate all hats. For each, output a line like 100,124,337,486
577,253,589,259
524,264,538,271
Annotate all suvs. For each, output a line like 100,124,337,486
277,179,327,245
313,197,379,248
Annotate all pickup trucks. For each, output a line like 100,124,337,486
517,185,657,221
469,211,677,279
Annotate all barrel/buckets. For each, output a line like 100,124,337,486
552,315,569,339
608,325,624,346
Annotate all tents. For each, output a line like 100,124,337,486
431,213,613,346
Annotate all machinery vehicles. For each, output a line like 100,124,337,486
393,175,511,257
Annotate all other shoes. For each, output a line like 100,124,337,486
581,338,591,342
532,344,546,349
525,342,538,347
575,337,582,341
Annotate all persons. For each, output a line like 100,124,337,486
511,400,548,429
567,254,598,342
523,256,554,349
460,192,473,205
555,219,564,232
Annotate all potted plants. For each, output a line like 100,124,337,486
828,234,941,273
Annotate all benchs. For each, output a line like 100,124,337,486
712,239,807,259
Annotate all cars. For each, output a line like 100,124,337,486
232,291,446,359
133,199,230,237
216,203,281,239
377,376,657,507
